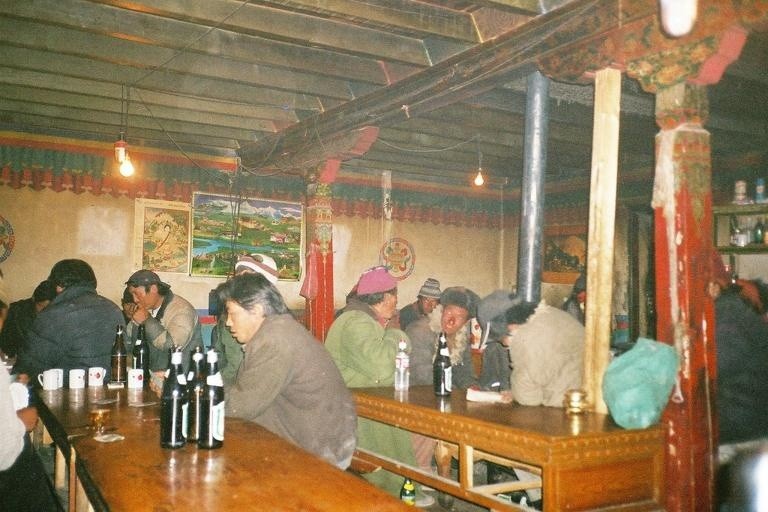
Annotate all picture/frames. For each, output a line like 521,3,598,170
540,226,587,283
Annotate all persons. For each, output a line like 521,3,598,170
216,273,358,473
0,362,38,470
208,253,278,390
403,285,482,470
0,256,205,386
712,246,768,446
334,274,585,392
477,290,585,511
323,269,436,508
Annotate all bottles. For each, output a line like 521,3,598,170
111,324,127,385
434,332,454,398
393,342,410,391
160,344,187,448
394,391,408,402
199,352,225,449
400,476,416,507
436,398,452,415
132,324,150,385
187,347,205,442
728,216,768,249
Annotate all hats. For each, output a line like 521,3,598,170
234,253,279,285
48,259,97,290
476,289,524,351
439,286,481,318
125,270,171,290
418,278,442,300
356,267,396,297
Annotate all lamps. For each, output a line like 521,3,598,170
118,156,135,177
474,171,484,186
113,142,127,163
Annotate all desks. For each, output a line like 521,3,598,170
23,381,425,512
349,384,667,511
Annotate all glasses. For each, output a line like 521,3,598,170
245,254,263,263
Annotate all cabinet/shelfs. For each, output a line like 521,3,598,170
707,200,768,253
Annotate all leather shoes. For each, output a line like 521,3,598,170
415,493,435,508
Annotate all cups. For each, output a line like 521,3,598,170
734,180,746,204
38,366,106,390
127,368,143,389
756,177,766,203
43,387,145,408
